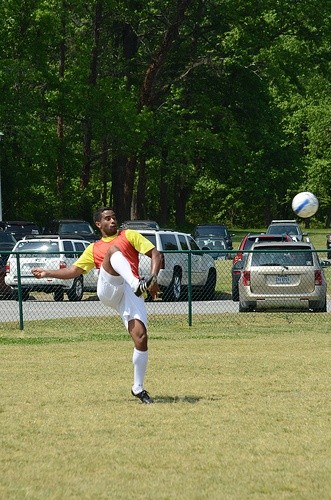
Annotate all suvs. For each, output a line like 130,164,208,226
4,235,99,301
238,239,327,313
117,227,217,300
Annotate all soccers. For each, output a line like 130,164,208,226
291,192,319,218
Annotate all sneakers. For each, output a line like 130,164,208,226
134,274,157,298
131,384,155,404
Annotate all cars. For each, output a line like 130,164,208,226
232,233,294,302
44,218,97,239
0,232,29,302
264,218,307,241
0,219,43,238
192,223,235,250
118,220,160,231
194,235,233,261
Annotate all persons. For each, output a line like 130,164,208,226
30,207,161,405
